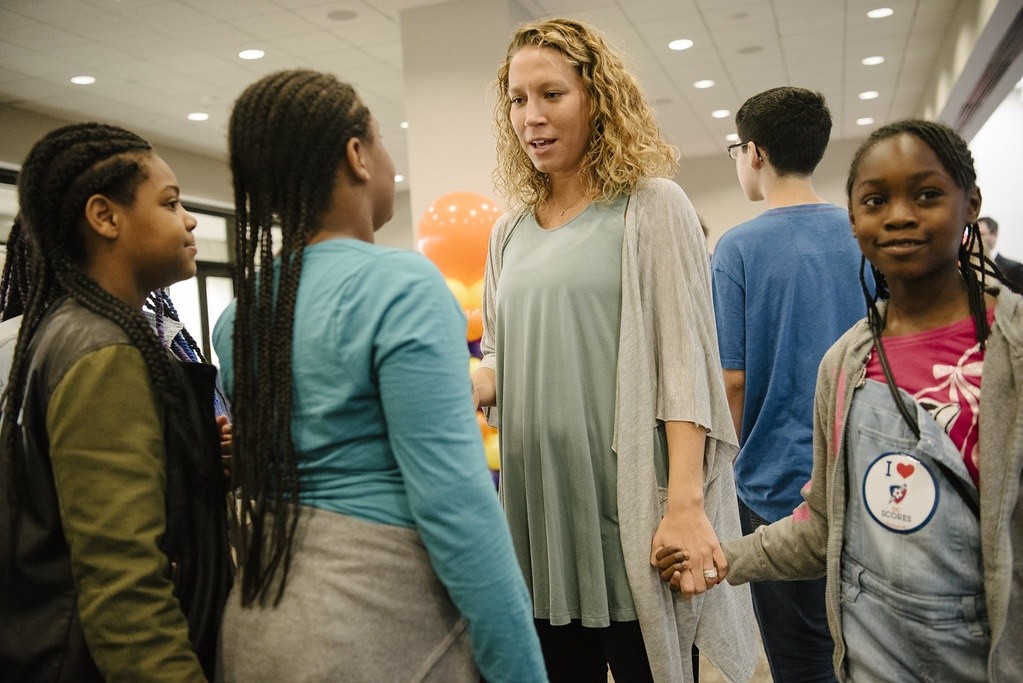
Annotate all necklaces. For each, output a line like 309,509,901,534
547,190,587,216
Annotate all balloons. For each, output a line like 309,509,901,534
418,190,506,492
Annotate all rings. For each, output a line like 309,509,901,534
704,567,718,578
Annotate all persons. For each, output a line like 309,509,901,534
469,17,730,683
0,209,228,420
211,67,552,683
656,121,1023,683
969,217,1023,296
710,84,891,683
0,124,235,683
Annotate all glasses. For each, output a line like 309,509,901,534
727,141,763,162
